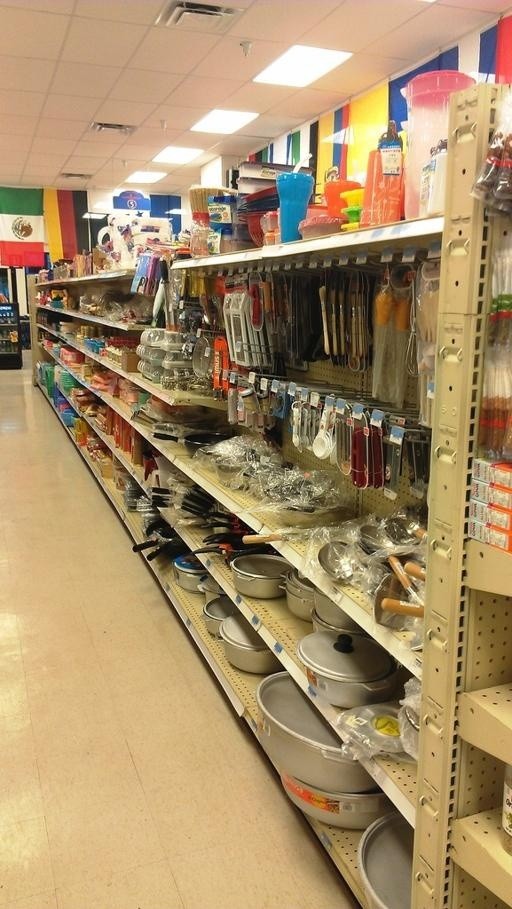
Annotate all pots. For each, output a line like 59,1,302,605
130,470,425,909
151,422,354,544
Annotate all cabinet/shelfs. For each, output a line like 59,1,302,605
27,81,512,909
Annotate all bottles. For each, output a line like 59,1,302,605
355,120,450,229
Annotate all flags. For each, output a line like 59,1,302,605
0,186,46,267
249,15,512,206
42,186,90,264
151,195,181,233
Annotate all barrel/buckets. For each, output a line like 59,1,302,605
398,71,481,220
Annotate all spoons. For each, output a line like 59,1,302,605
318,508,428,662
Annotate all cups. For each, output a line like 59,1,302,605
274,172,317,244
